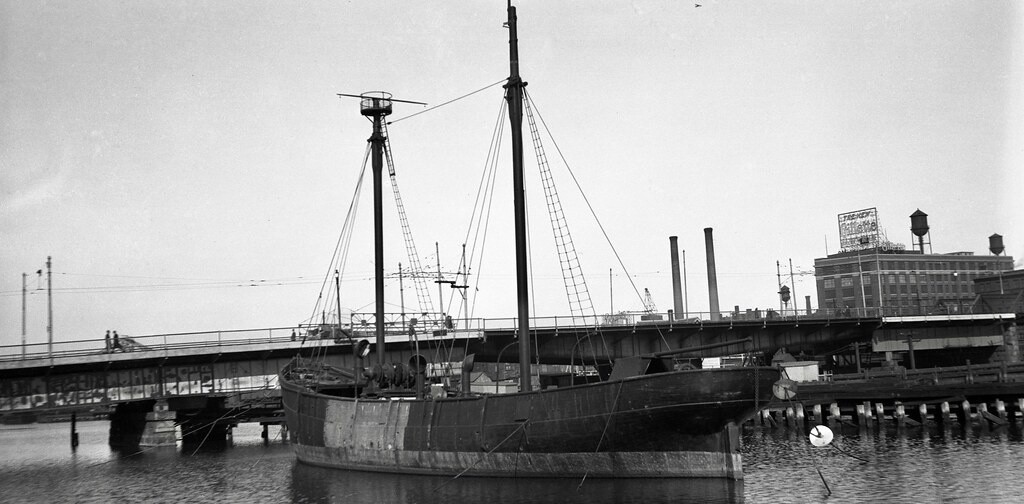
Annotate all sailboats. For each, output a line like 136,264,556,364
278,0,868,495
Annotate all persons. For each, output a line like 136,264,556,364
110,331,125,354
843,305,851,317
835,305,841,317
291,329,296,341
755,308,760,318
104,330,112,347
